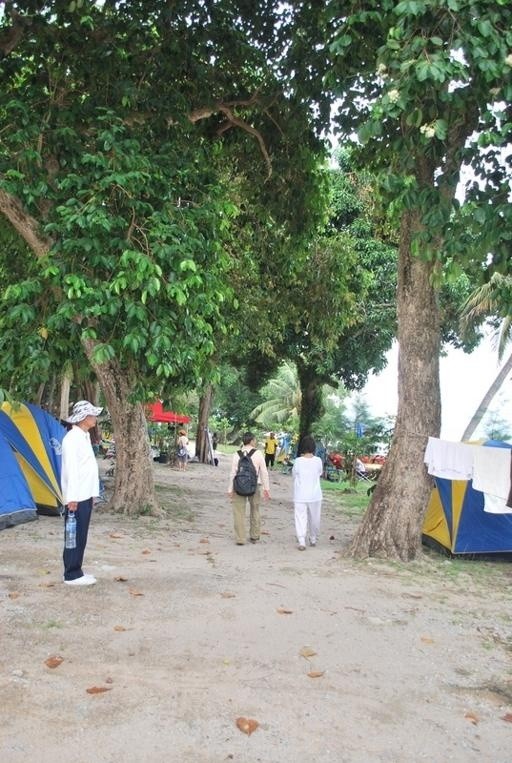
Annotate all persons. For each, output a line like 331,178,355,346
58,397,105,586
355,456,366,477
264,432,278,473
176,429,189,471
212,431,219,451
227,432,272,545
291,435,323,551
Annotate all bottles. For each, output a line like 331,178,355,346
65,512,77,549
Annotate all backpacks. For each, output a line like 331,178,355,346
233,448,259,496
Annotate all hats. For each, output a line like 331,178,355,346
62,398,106,424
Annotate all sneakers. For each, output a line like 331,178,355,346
62,572,98,586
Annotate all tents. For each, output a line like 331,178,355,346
0,399,107,531
422,437,512,563
